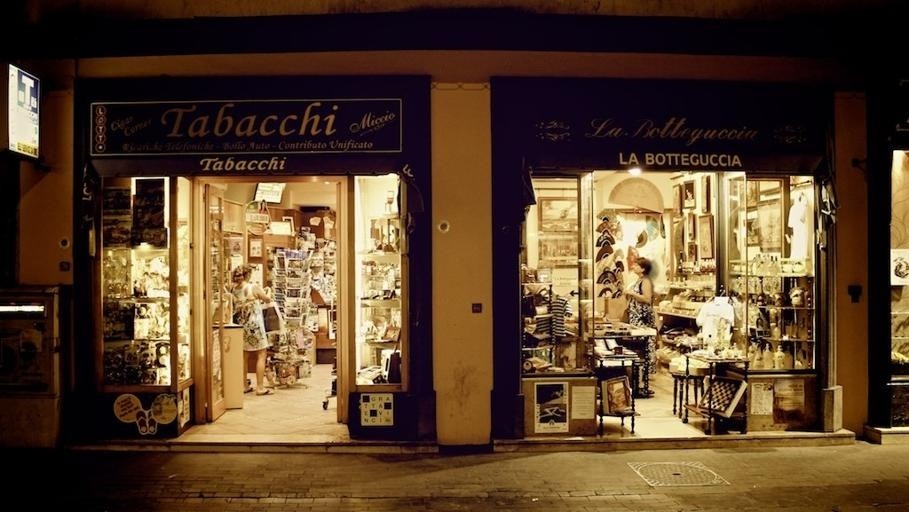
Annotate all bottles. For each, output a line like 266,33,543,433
753,343,786,369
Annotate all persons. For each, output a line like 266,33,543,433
229,263,279,396
625,256,660,391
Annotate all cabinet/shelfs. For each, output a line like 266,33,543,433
522,283,554,357
653,308,701,366
348,173,410,439
736,272,815,374
102,177,195,440
272,251,312,319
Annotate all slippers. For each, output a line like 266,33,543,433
243,387,255,394
255,390,275,396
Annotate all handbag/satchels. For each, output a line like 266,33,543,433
620,308,632,323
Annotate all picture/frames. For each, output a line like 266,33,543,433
734,180,782,248
537,197,580,268
248,235,264,260
673,175,713,263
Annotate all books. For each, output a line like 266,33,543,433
272,246,312,386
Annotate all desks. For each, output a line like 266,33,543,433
584,323,654,396
213,324,244,409
585,350,641,435
683,353,750,435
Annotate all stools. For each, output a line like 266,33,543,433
672,371,705,419
